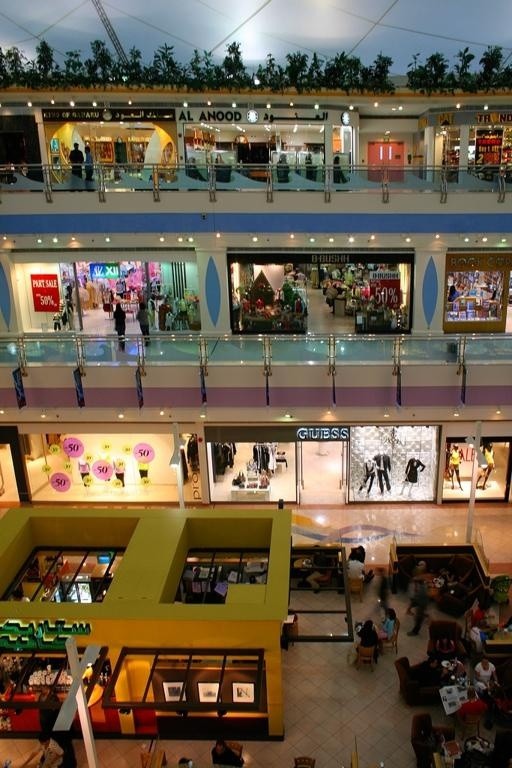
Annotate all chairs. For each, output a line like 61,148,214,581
348,575,365,602
315,558,335,588
295,756,315,767
394,554,511,768
354,610,400,671
224,741,243,762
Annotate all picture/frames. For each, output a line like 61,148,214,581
232,680,256,703
197,680,222,703
162,679,187,702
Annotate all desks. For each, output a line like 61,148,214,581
293,556,312,588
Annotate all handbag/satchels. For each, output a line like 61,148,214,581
232,460,257,485
444,472,452,481
479,468,485,477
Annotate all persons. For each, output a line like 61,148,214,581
356,450,425,497
77,454,149,488
305,153,313,180
69,142,93,192
354,607,398,666
404,556,463,637
21,684,64,768
429,590,511,767
276,154,289,183
451,441,495,491
178,736,242,768
12,591,30,603
334,156,347,183
65,296,75,330
448,286,460,301
187,154,231,183
162,142,173,166
305,546,375,593
113,302,152,352
